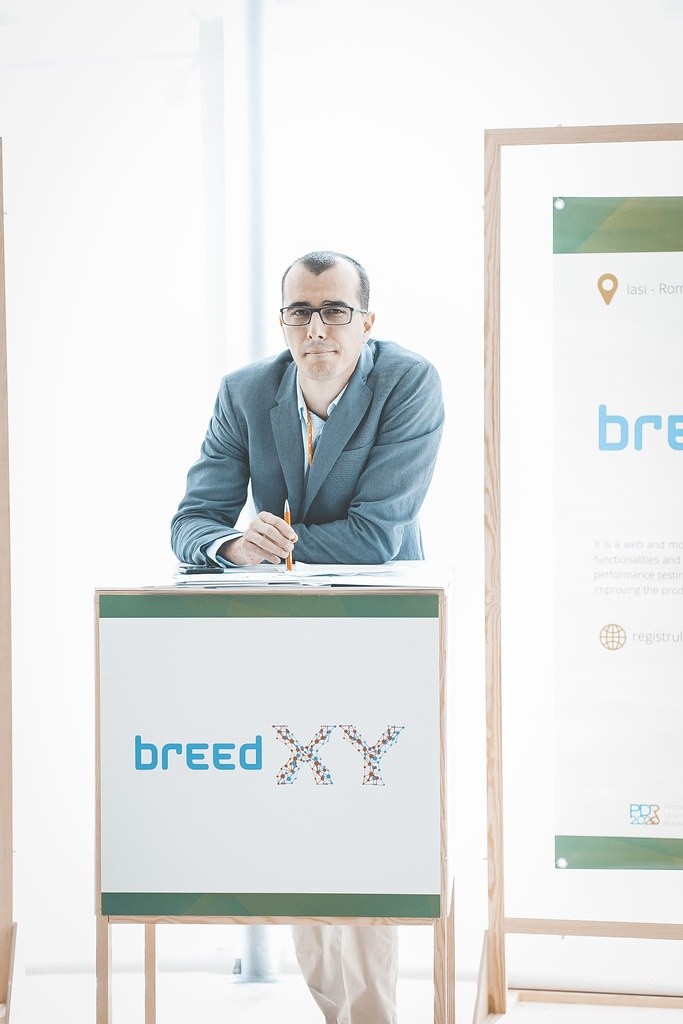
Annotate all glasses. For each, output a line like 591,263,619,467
279,304,368,326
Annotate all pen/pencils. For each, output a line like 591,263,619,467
284,498,293,571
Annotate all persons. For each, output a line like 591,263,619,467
169,253,446,1024
